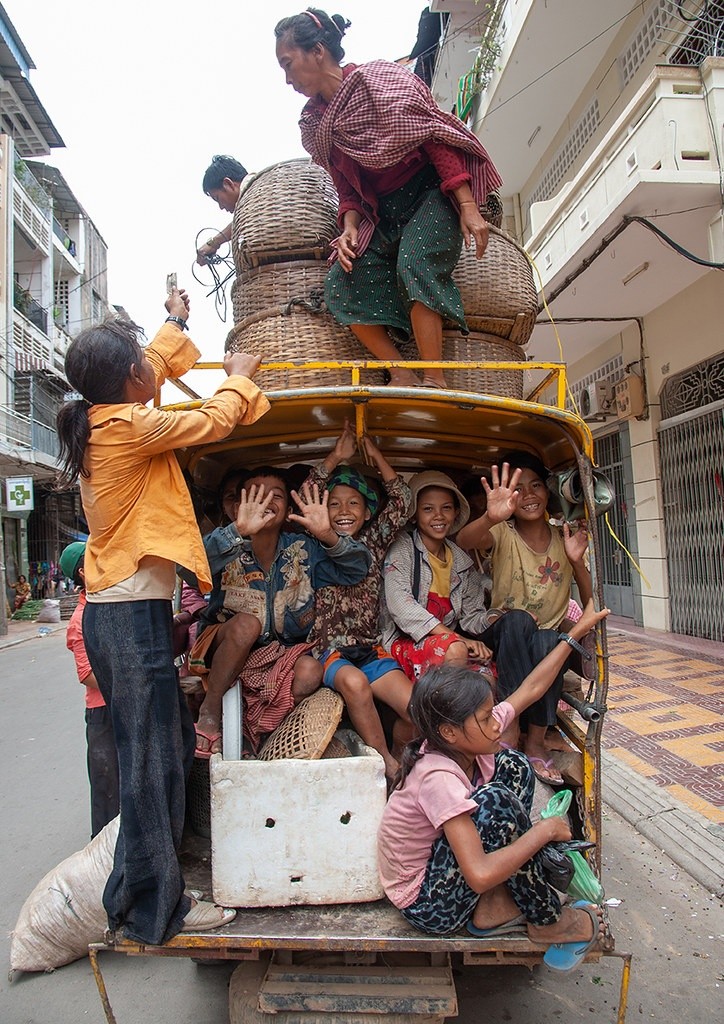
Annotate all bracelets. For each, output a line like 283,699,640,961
166,314,193,333
206,237,223,250
554,630,593,663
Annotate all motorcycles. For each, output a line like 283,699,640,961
87,358,633,1024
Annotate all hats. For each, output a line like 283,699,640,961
59,542,86,580
408,470,471,537
324,465,380,527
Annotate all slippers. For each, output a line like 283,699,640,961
192,723,223,759
527,756,564,785
544,900,602,974
466,913,527,936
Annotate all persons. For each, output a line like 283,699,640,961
297,420,423,780
54,539,118,838
274,3,504,387
8,573,34,609
191,153,261,267
168,419,594,788
54,285,272,950
378,597,612,974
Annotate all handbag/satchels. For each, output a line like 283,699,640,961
534,840,597,893
540,790,605,904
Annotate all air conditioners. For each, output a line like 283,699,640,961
577,379,617,420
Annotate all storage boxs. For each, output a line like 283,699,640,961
210,728,388,906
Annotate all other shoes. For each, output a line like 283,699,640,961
183,889,203,899
181,898,236,931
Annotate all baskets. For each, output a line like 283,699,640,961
392,329,526,400
443,221,538,345
258,688,352,761
224,303,385,392
232,157,344,278
230,260,336,326
